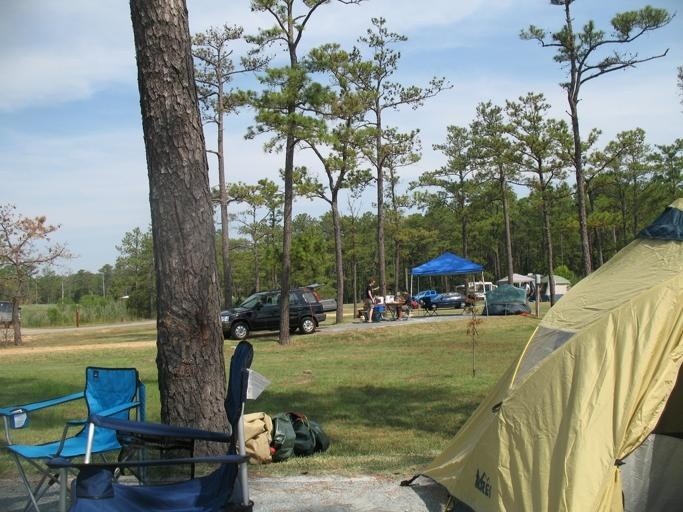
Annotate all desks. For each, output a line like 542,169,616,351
386,302,405,321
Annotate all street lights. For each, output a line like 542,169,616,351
96,271,105,298
59,273,68,304
33,276,41,305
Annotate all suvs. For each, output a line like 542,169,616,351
220,283,326,340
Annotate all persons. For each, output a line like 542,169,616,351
397,288,411,319
465,295,473,307
365,278,380,322
526,282,530,304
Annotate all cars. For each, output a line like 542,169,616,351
430,292,474,310
0,300,20,327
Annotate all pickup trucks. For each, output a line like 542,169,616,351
410,290,441,305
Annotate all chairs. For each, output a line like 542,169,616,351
0,366,146,512
48,338,255,512
419,297,438,316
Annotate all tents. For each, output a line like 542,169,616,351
482,283,531,315
542,274,571,302
401,197,683,511
